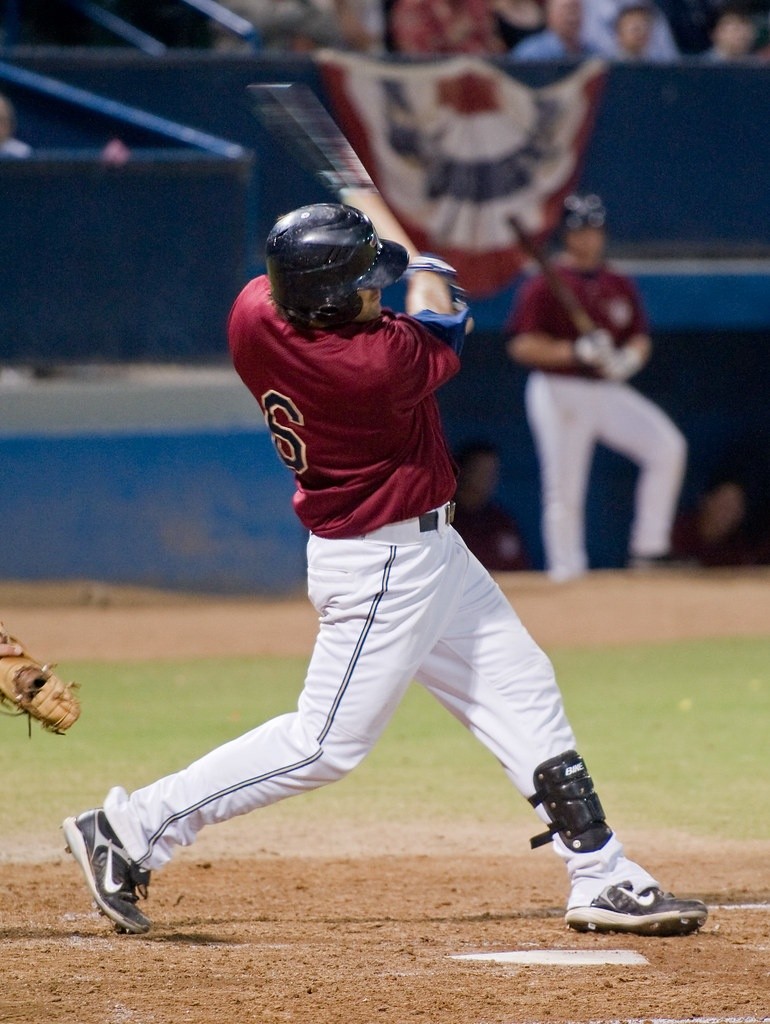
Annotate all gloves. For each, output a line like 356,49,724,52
575,328,615,366
601,349,641,382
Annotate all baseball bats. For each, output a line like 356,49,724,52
261,76,418,267
505,211,602,335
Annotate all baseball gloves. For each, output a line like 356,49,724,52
0,628,83,741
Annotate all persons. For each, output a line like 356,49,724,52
207,0,770,63
61,190,708,936
450,193,756,582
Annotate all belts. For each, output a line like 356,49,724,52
419,502,456,532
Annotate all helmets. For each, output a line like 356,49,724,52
266,202,409,315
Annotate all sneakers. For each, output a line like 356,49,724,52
565,881,707,937
62,808,153,934
